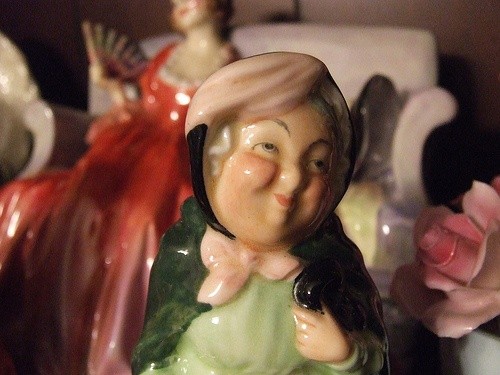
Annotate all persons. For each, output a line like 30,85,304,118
0,1,241,375
131,51,391,374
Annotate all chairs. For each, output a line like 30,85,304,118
88,19,459,298
